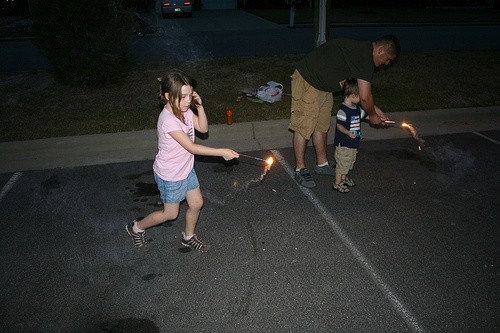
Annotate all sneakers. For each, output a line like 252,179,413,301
181,232,210,253
125,219,147,249
313,160,336,176
294,167,317,188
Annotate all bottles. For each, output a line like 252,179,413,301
226,106,234,125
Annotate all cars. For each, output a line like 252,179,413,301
160,0,193,18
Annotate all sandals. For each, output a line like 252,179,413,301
343,178,356,187
332,181,350,193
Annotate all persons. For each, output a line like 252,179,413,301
288,34,401,189
125,70,240,252
332,78,391,194
